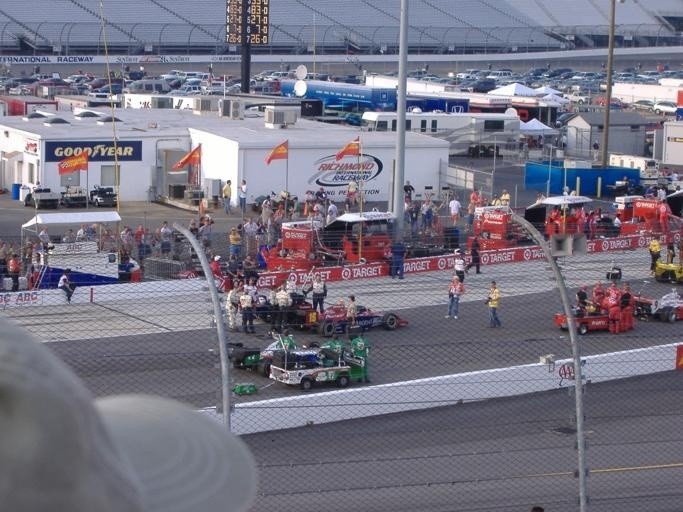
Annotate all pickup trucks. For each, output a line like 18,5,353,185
562,89,593,107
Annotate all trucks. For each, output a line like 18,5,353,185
357,113,521,157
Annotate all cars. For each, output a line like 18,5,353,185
632,99,655,112
597,97,630,109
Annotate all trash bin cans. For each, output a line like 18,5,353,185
19,185,30,202
11,184,22,200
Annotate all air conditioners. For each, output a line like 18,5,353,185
229,100,246,121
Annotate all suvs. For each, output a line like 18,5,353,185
650,101,681,115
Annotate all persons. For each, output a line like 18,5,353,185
593,139,599,162
487,280,501,328
209,252,357,334
207,167,511,283
662,287,681,306
444,276,465,319
523,142,529,161
0,212,214,303
536,176,683,275
561,134,566,149
0,317,260,512
575,279,640,334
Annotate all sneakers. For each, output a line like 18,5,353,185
444,314,458,320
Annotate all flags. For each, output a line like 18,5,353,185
172,145,201,169
58,151,88,176
264,140,288,166
336,136,359,161
